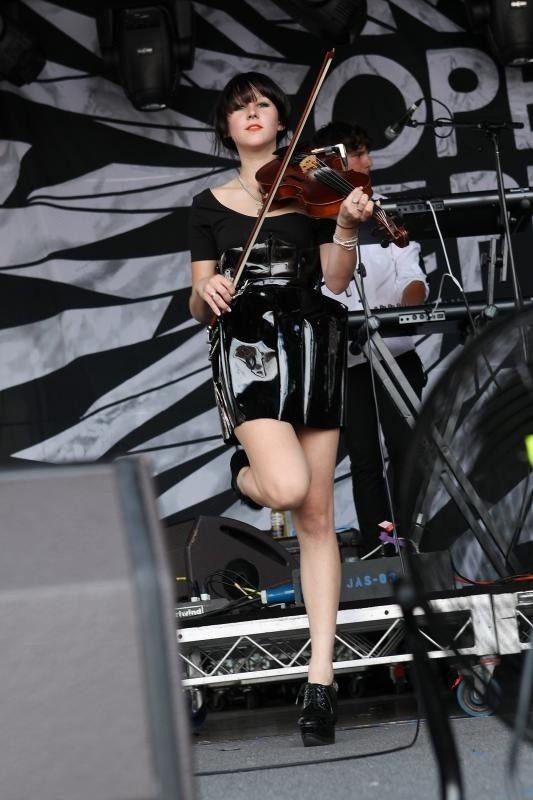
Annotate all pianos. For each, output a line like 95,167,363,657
345,187,533,581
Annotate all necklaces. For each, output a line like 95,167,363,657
238,175,272,217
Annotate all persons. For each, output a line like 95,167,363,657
189,71,381,747
312,120,430,561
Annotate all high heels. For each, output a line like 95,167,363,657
295,683,338,747
230,450,263,510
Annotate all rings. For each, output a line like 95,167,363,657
352,200,358,204
357,205,364,212
211,292,218,299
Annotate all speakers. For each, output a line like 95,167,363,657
163,513,300,600
0,458,196,800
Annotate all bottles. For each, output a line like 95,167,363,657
261,584,295,605
271,509,288,539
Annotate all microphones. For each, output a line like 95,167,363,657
382,96,423,141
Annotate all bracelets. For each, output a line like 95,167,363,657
336,224,358,229
333,230,358,250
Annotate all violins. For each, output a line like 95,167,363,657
255,142,410,248
